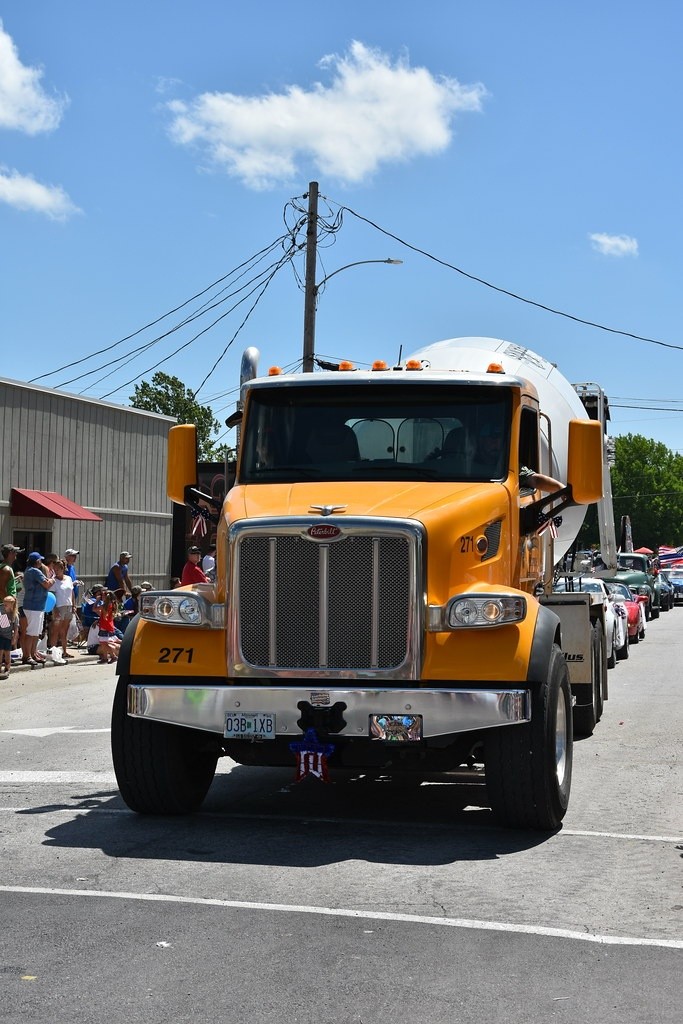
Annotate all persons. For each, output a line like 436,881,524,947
0,544,80,679
169,577,181,588
81,551,155,664
202,544,217,575
422,417,568,502
181,546,211,586
256,426,306,483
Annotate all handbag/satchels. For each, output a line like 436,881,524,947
66,613,79,641
36,634,66,664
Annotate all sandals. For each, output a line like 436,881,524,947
62,653,74,658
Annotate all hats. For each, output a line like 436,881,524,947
1,544,20,555
189,545,201,554
120,551,133,558
141,581,154,590
28,552,44,561
132,585,146,594
65,548,80,557
92,584,108,593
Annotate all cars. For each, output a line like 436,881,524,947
548,552,683,669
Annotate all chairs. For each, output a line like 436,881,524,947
440,425,500,474
289,423,360,469
74,606,99,648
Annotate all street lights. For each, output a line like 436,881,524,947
302,258,404,373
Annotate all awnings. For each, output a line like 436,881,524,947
9,488,103,521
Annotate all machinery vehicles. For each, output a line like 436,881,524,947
110,336,618,832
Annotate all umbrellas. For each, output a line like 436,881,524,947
634,547,654,553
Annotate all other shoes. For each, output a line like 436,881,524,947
0,673,9,680
97,659,108,664
108,655,118,664
22,657,37,665
2,654,14,663
32,652,46,663
5,667,10,673
